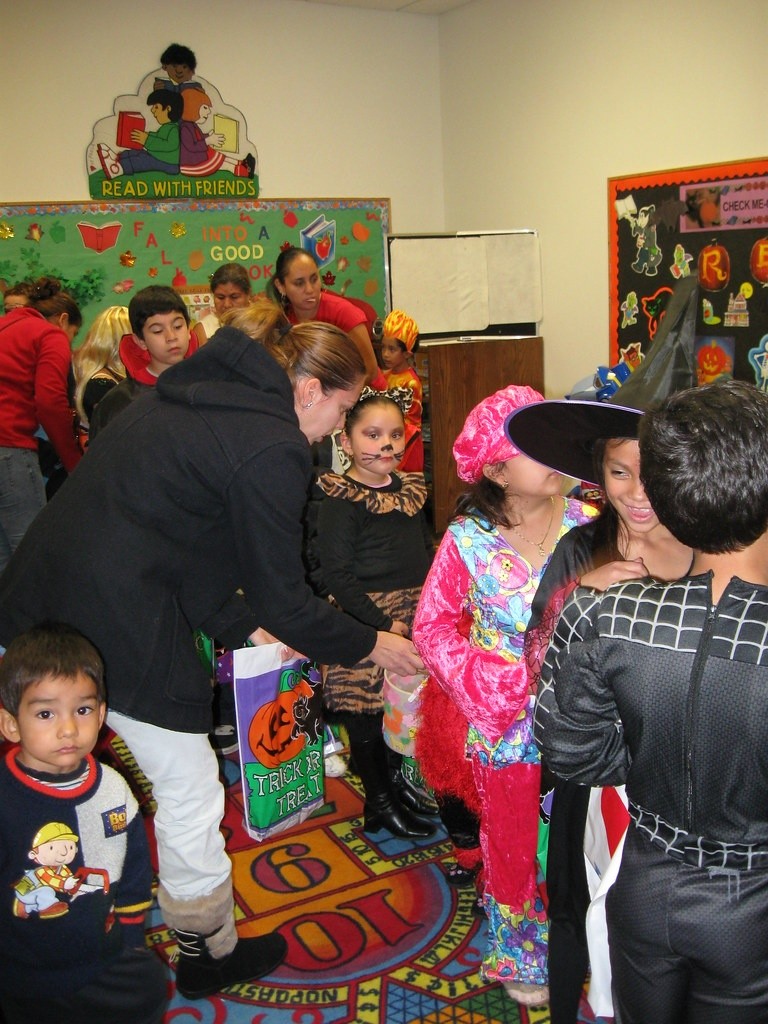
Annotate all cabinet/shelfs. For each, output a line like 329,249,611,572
369,337,545,538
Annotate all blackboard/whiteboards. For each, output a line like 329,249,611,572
606,157,768,397
383,229,543,343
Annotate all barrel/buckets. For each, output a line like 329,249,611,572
381,669,428,757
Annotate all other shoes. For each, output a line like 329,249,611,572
502,976,551,1004
469,891,490,920
445,861,482,888
323,753,349,777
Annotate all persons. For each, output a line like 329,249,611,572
0,245,436,999
412,378,768,1024
0,621,173,1023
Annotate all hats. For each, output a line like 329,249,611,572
382,309,419,356
453,384,545,485
504,271,704,486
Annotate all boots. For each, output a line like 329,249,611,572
387,765,439,815
157,870,289,999
363,778,436,840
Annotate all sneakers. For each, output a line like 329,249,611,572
210,724,239,754
